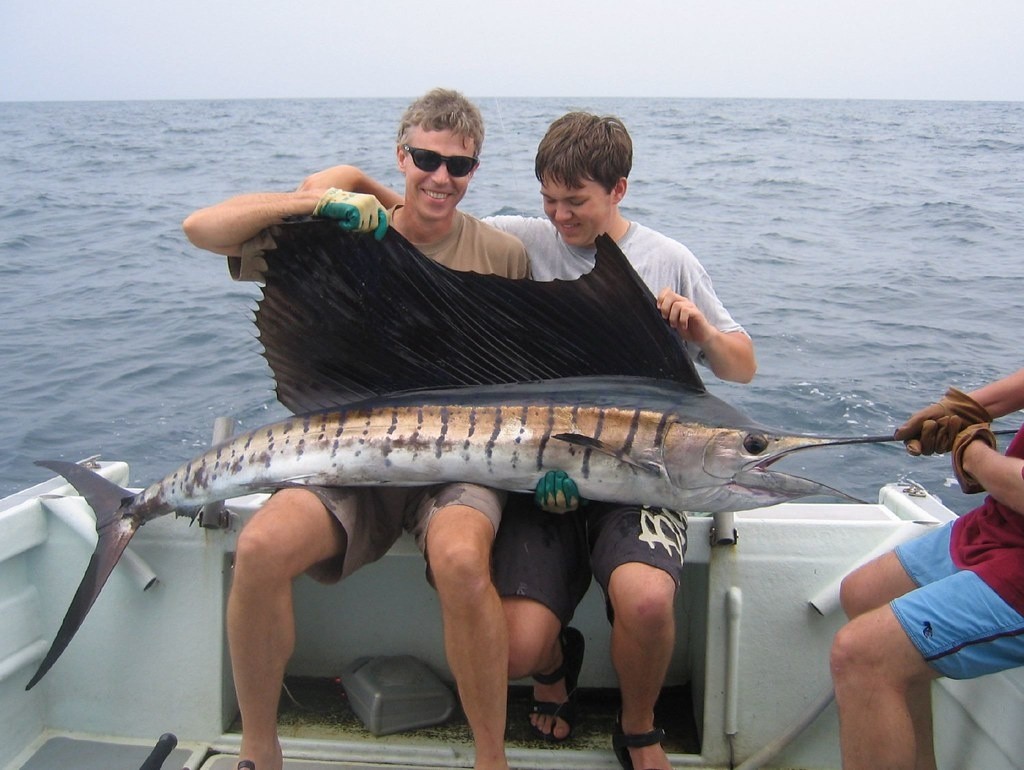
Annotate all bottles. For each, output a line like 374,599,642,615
341,655,453,736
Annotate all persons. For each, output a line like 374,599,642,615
182,89,758,770
831,367,1024,770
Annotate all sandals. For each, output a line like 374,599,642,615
529,626,585,739
612,711,665,770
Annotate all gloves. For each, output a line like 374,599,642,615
533,470,580,513
892,388,996,495
402,144,477,177
313,187,389,241
